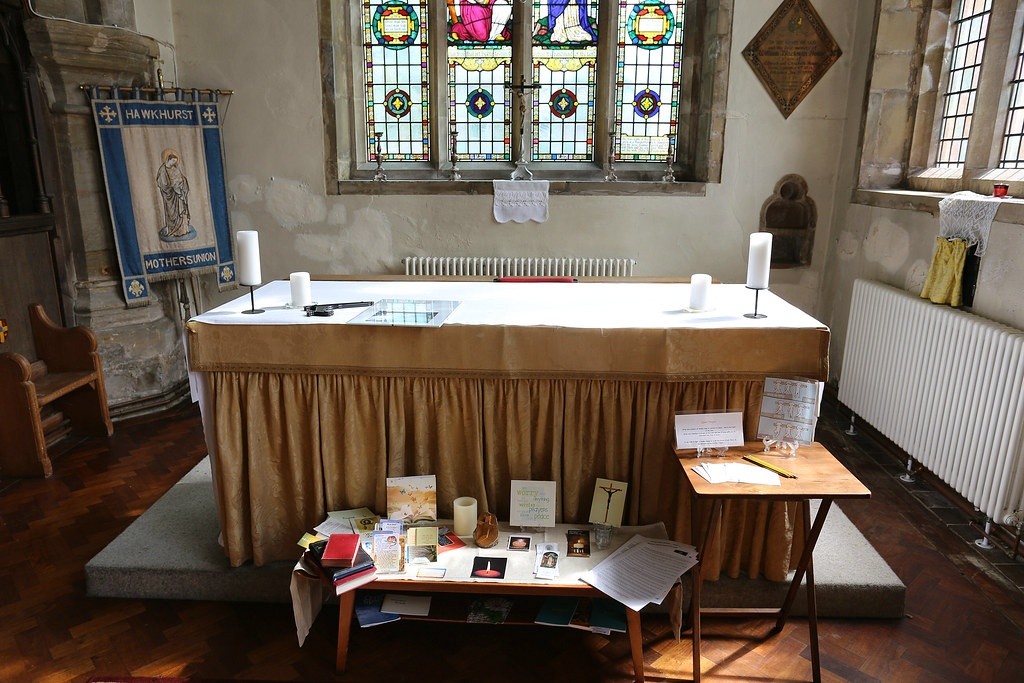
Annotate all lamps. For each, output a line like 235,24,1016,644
290,272,310,306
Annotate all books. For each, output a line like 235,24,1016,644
355,590,432,627
466,595,514,624
533,598,627,635
304,534,378,597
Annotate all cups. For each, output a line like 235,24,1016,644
290,272,309,306
594,522,615,550
689,274,712,312
994,185,1009,197
453,496,477,536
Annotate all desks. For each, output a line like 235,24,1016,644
674,440,873,683
289,516,685,683
182,282,833,582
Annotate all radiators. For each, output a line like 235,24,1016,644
838,276,1024,550
400,257,636,277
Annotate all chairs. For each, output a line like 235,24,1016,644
0,304,122,479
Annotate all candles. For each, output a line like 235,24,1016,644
746,232,772,288
689,274,713,310
236,230,262,285
